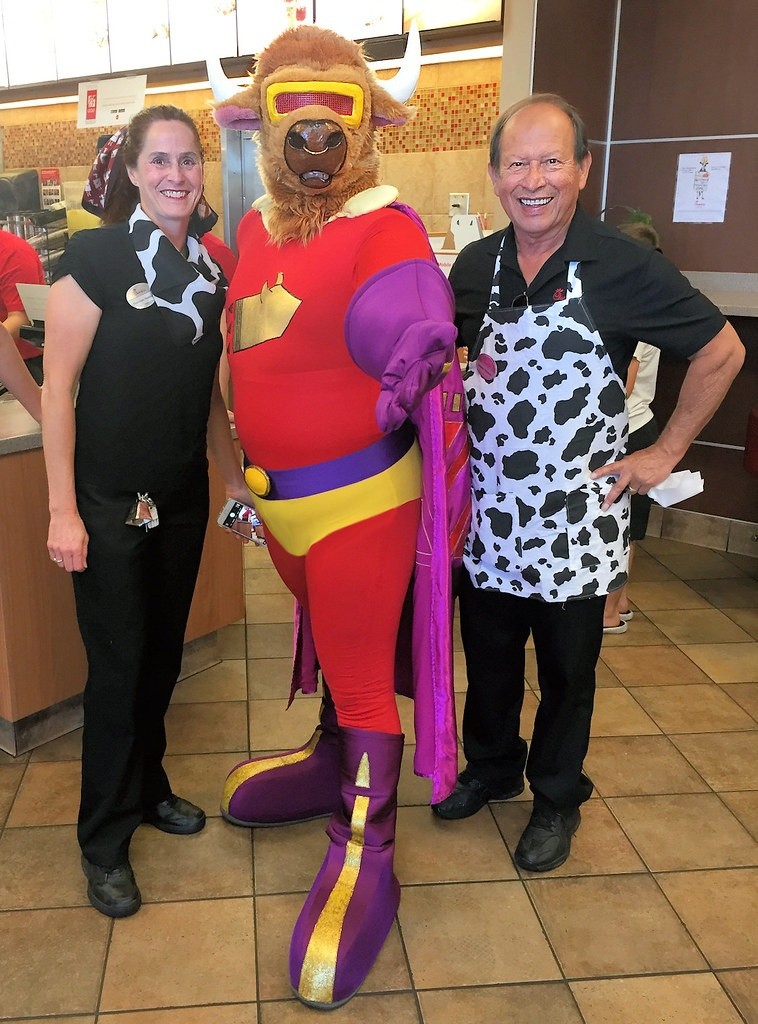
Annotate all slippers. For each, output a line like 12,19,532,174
619,609,633,621
603,619,627,634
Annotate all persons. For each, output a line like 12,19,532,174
602,223,661,634
431,93,745,873
0,231,237,427
39,105,230,922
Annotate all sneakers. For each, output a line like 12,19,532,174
81,853,142,918
514,801,581,873
140,794,206,835
430,763,524,820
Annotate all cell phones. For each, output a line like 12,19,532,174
217,497,268,546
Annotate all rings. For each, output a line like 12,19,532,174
56,559,62,562
627,485,637,492
51,558,56,561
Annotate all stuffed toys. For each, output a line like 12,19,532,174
216,27,475,1010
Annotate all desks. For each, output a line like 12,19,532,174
0,394,244,756
